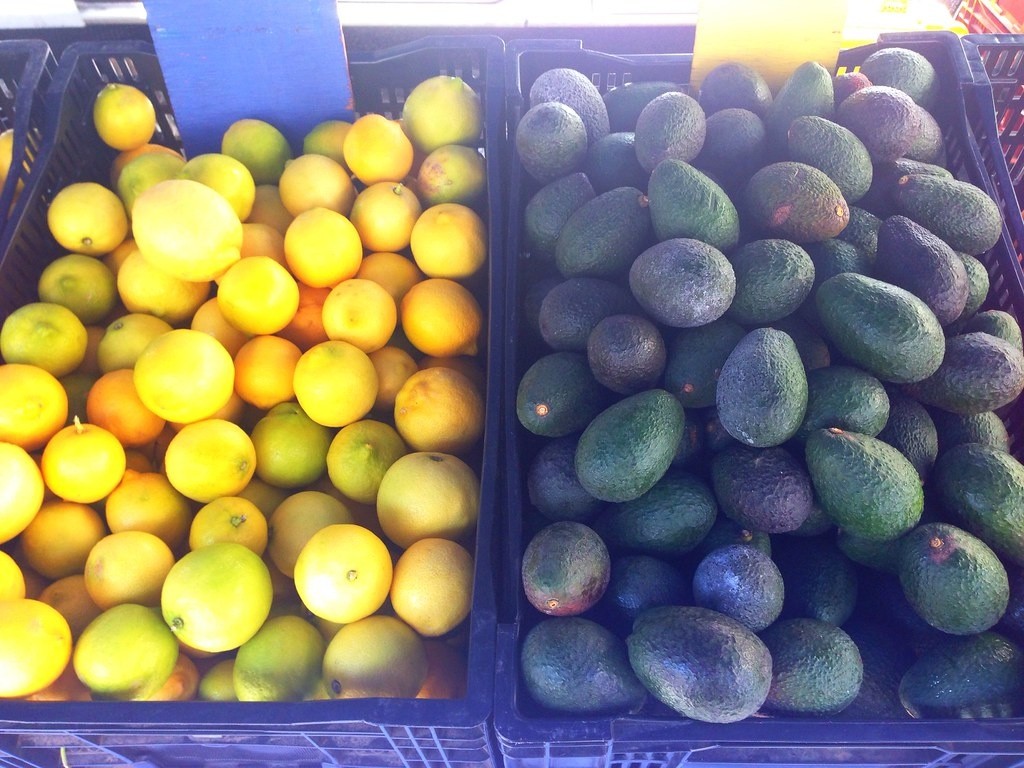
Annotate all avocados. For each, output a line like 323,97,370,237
517,48,1024,727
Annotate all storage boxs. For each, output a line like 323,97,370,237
0,32,511,768
0,37,60,230
494,30,1024,768
962,35,1024,258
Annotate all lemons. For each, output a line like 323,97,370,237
0,73,488,701
